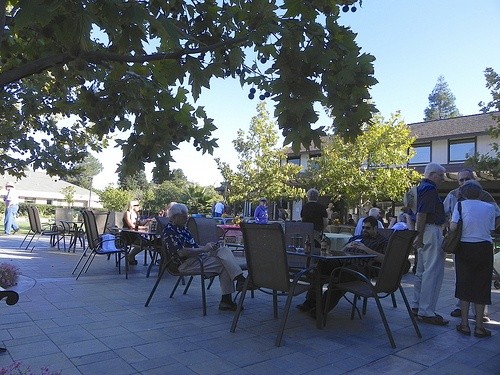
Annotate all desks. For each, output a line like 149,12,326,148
123,229,162,266
226,241,378,329
60,220,85,247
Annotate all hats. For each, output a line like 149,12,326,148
5,182,14,187
404,186,417,214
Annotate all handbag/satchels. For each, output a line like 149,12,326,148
442,201,463,253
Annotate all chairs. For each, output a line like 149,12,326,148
20,204,423,350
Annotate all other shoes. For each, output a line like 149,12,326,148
451,309,461,316
219,303,243,311
236,280,258,291
311,308,318,319
296,300,314,310
420,312,449,325
411,309,418,318
13,228,19,235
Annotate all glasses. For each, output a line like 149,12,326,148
134,204,139,206
458,178,471,182
362,226,373,230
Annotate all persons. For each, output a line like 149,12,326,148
121,200,177,264
325,203,356,226
3,182,20,235
214,201,224,217
300,189,328,265
407,163,449,325
164,204,259,311
296,217,388,317
277,204,288,221
387,206,418,272
443,168,500,321
254,199,268,225
450,180,495,335
354,208,384,235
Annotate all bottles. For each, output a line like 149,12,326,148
304,233,311,254
321,233,327,252
135,222,138,231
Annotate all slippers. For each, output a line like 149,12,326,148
475,327,491,336
457,324,471,335
